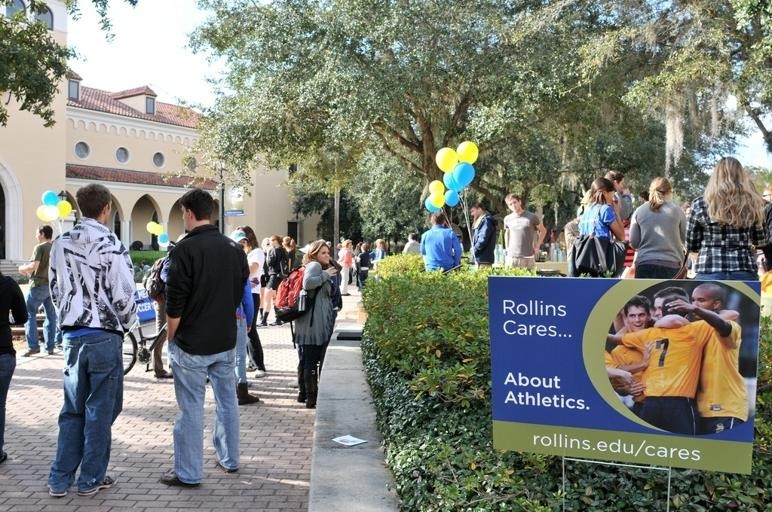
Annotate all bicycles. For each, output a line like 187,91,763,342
119,308,179,376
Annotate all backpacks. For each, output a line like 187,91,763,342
273,264,312,322
141,258,165,299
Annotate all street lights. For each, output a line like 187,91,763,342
215,150,226,234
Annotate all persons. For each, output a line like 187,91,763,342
48,183,137,497
231,225,388,408
567,157,772,281
401,193,547,272
159,189,250,486
20,225,56,357
604,282,750,435
1,270,28,463
140,240,176,379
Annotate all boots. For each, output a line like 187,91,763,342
236,381,260,405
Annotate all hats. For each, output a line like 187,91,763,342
230,230,249,244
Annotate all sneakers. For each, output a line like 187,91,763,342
269,320,281,328
305,402,316,409
47,484,68,497
298,392,306,403
77,475,114,495
255,369,265,378
281,320,290,327
215,462,240,473
245,366,257,371
21,348,40,358
160,474,200,488
44,347,54,356
256,320,268,328
155,371,173,378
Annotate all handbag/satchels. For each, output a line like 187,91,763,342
571,233,630,276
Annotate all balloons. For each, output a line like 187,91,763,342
37,191,71,222
147,222,169,247
425,140,479,213
